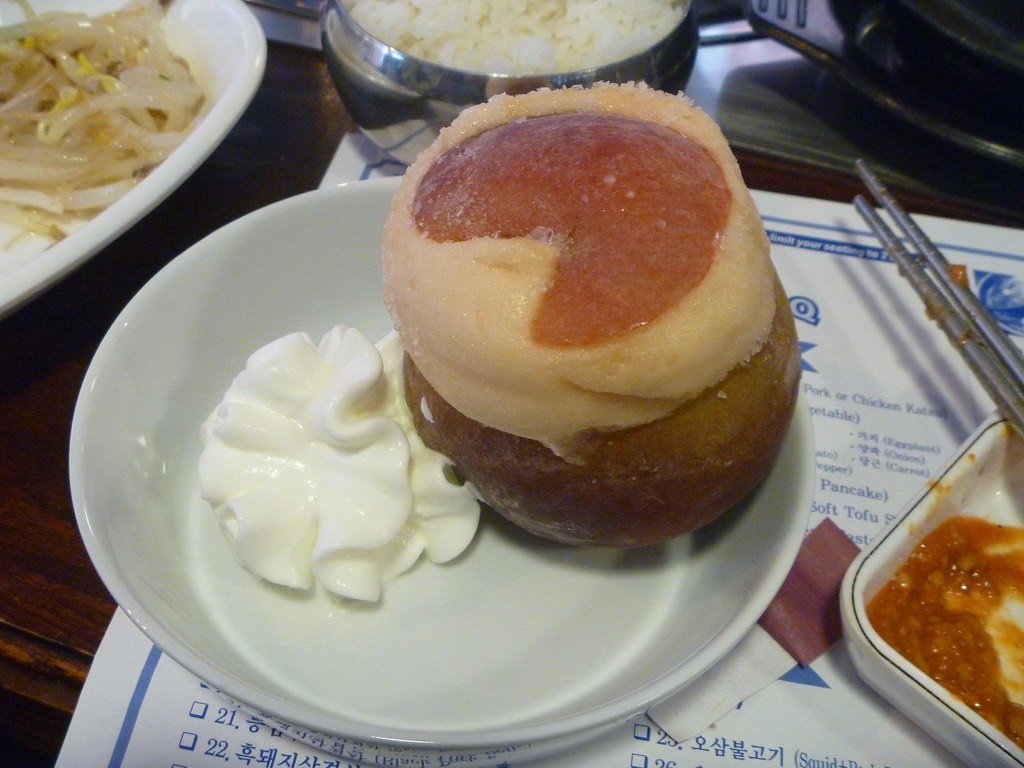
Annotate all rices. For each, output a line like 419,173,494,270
338,0,684,75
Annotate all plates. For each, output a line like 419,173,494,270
842,395,1021,767
1,1,265,319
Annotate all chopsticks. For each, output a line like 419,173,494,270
848,156,1024,444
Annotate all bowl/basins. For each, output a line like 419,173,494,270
70,175,816,744
319,4,700,172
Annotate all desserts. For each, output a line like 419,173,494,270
381,80,801,551
